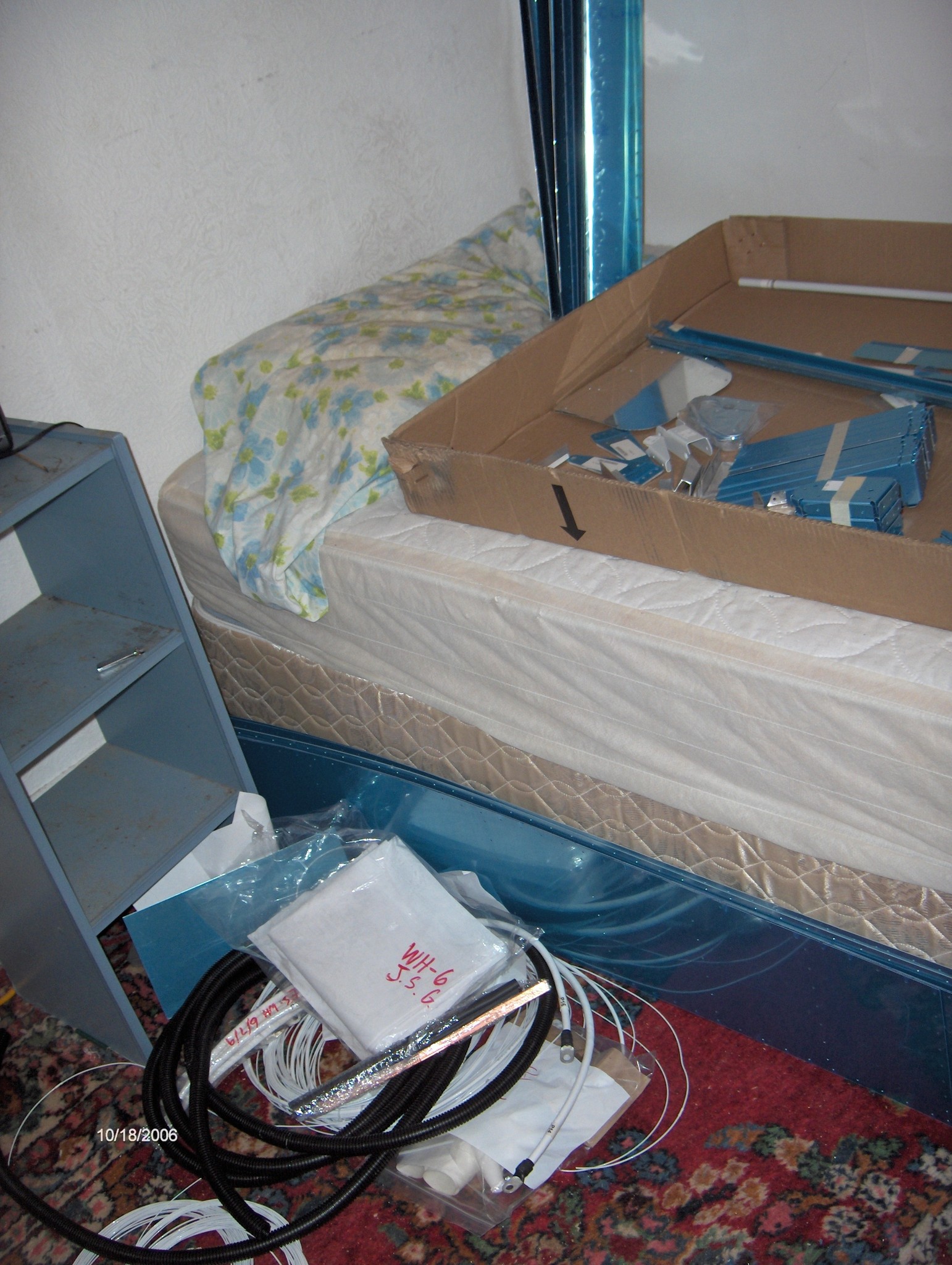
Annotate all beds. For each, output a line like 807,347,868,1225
157,246,952,1124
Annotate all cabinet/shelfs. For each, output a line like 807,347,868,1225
0,418,259,1068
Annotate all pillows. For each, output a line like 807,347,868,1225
189,185,670,623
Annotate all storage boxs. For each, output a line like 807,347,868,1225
379,215,951,630
